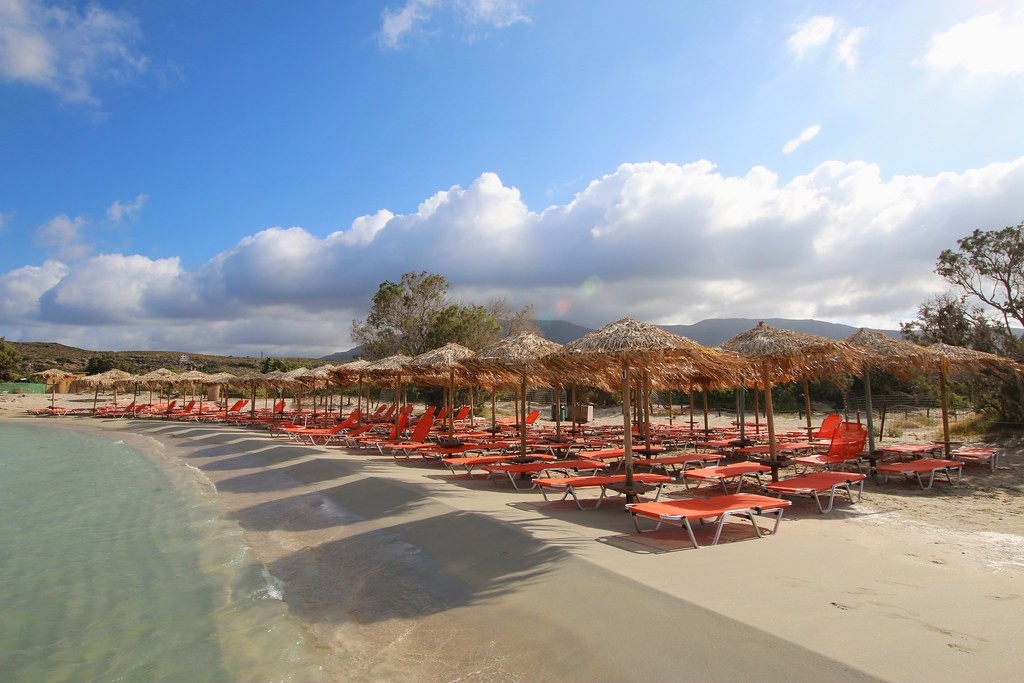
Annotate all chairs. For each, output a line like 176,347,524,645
26,401,1008,549
